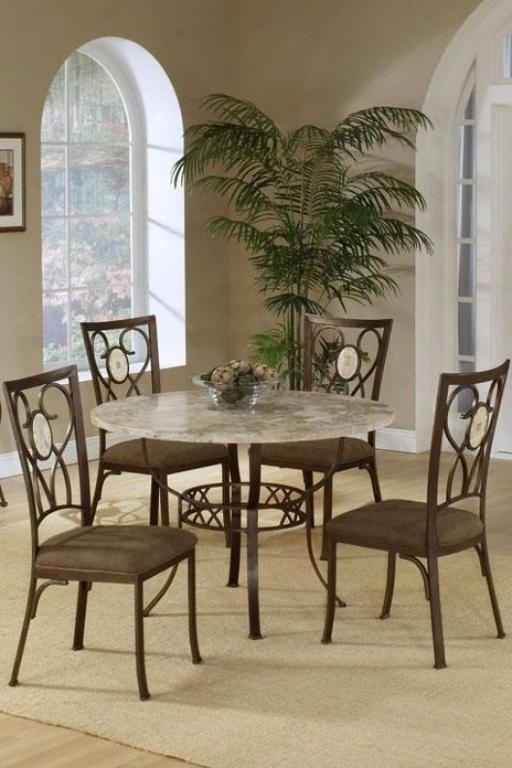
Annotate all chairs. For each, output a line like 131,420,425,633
5,365,204,700
248,314,392,559
319,358,509,669
82,314,235,550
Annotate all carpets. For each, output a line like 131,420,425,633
3,454,512,768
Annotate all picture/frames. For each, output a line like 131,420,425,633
0,131,26,234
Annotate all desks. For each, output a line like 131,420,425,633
92,390,396,639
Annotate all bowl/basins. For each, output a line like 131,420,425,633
191,376,281,411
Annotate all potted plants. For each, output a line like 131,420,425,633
173,92,433,395
